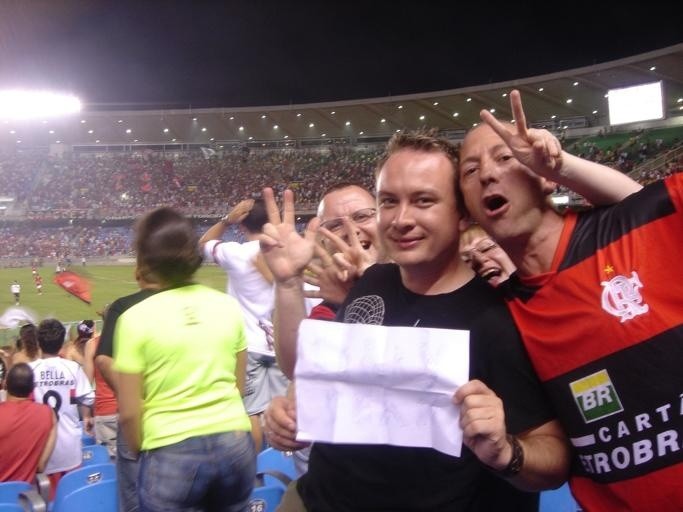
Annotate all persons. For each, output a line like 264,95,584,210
1,88,683,512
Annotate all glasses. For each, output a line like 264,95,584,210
320,208,376,233
